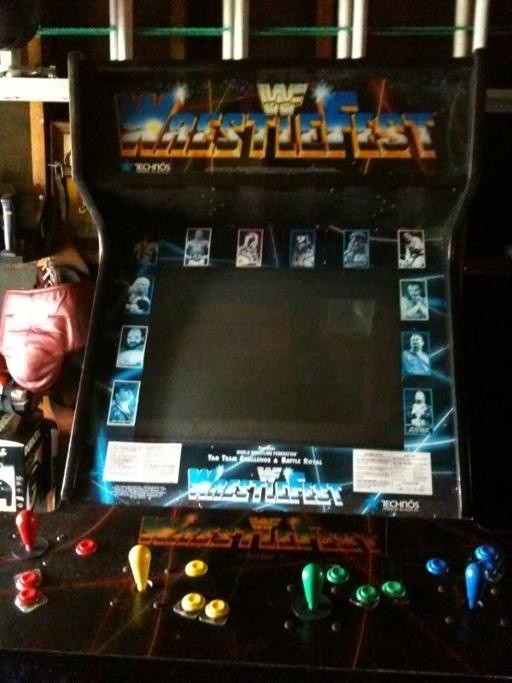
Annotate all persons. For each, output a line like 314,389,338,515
402,388,433,432
120,326,145,369
124,273,152,317
399,230,425,269
110,382,137,425
343,230,370,270
401,332,429,377
183,228,210,265
401,281,429,321
237,231,261,268
290,231,315,266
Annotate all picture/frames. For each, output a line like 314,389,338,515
49,120,100,250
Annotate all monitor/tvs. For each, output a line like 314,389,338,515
93,223,463,516
